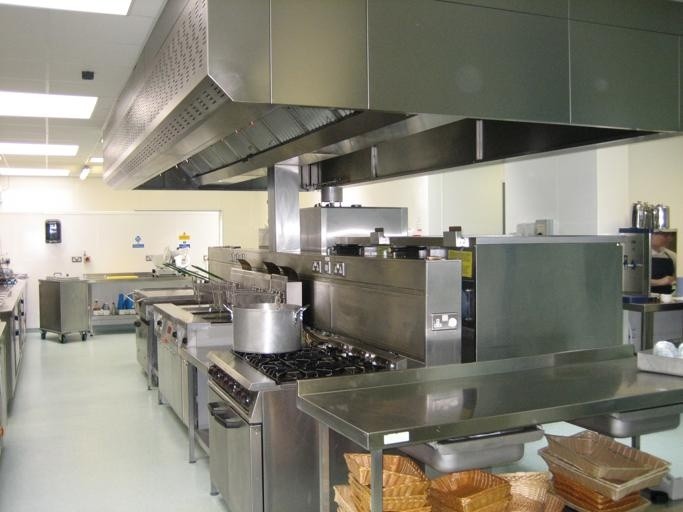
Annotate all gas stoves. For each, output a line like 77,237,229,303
205,336,409,426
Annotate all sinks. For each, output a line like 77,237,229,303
202,316,229,319
190,310,229,314
209,320,232,323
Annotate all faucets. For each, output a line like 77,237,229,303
209,306,226,312
220,302,234,322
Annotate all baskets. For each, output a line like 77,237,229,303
537,429,672,512
427,468,513,512
333,452,432,512
496,471,565,512
333,429,672,512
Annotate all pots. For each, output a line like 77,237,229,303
221,299,312,356
